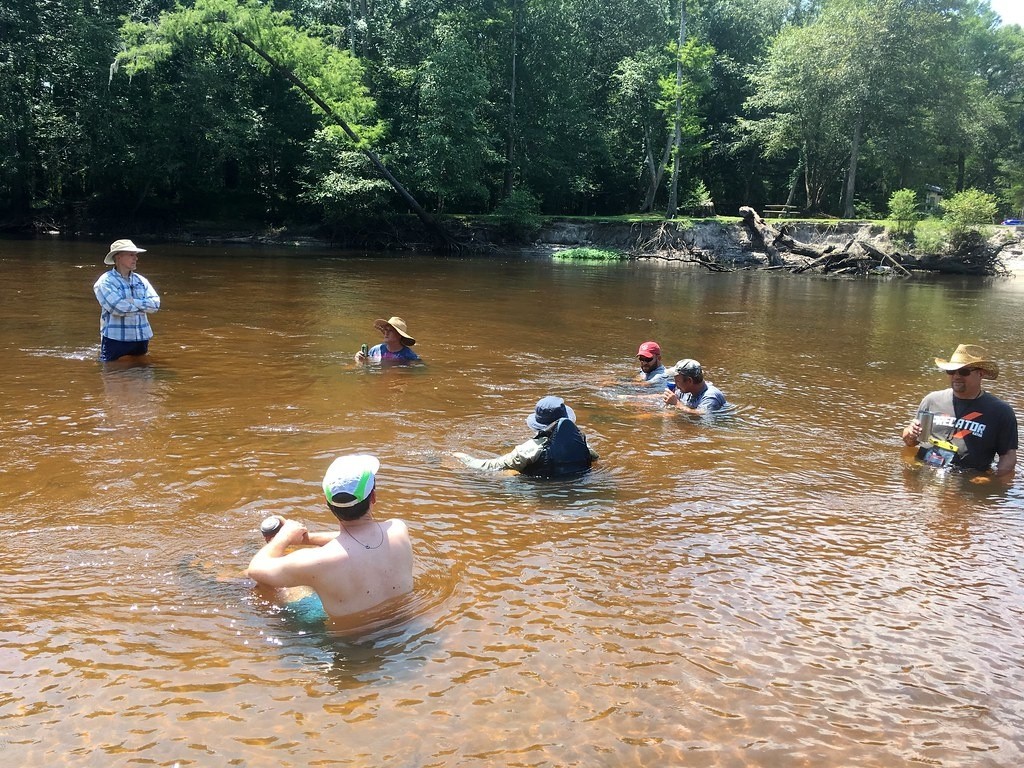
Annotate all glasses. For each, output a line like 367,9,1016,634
639,355,658,363
946,368,979,377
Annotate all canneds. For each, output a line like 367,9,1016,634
361,343,368,357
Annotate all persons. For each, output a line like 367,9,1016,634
426,392,601,498
899,342,1019,486
181,451,419,669
567,341,739,431
92,239,161,361
355,316,425,373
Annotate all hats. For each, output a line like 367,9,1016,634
635,341,660,358
322,454,381,507
665,358,701,377
373,316,416,346
526,396,576,432
104,239,147,265
935,343,999,380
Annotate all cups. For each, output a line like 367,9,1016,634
915,410,934,442
260,518,282,544
667,382,676,394
